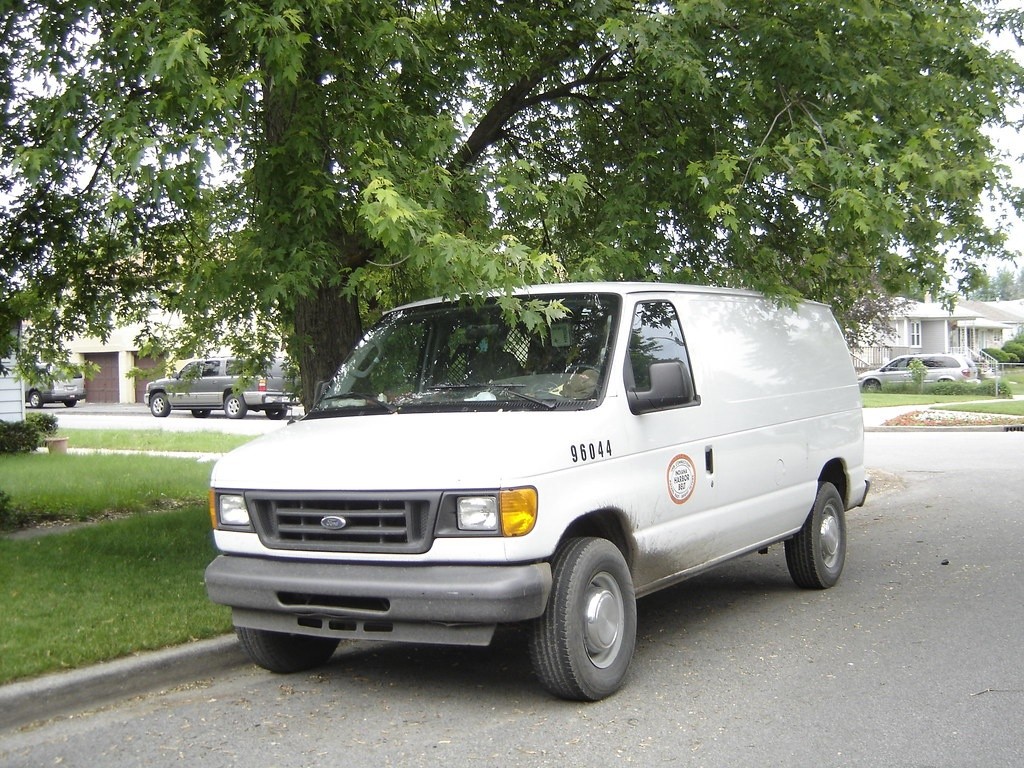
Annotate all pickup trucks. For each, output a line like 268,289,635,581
143,355,302,420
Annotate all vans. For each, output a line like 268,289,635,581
198,280,871,702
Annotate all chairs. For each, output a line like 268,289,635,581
494,353,525,380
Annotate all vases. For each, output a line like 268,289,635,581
45,437,69,453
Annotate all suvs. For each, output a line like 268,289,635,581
857,352,982,395
23,362,87,409
